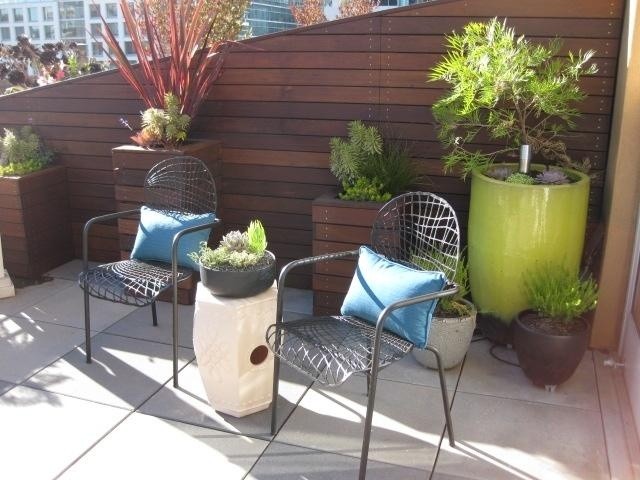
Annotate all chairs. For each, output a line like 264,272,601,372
265,190,459,480
78,156,224,388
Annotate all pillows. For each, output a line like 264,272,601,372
339,245,447,349
130,206,216,271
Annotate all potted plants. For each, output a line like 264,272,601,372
426,15,600,348
311,119,436,314
187,220,277,298
410,245,477,370
0,124,74,279
513,259,602,388
84,0,264,307
